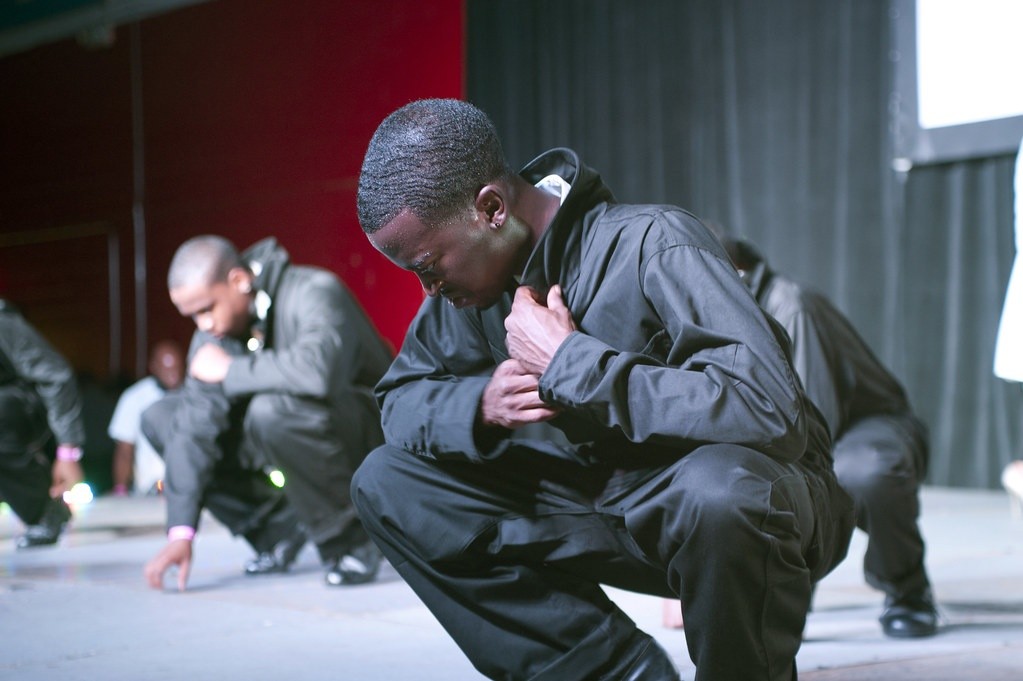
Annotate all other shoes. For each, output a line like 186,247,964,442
245,521,311,577
599,636,679,681
23,499,72,545
326,533,384,584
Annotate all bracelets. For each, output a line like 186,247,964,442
112,486,128,496
169,526,194,542
57,447,80,462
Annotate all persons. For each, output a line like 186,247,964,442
0,304,85,548
108,345,186,497
663,239,937,638
351,100,856,681
145,236,394,591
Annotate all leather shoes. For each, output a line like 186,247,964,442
878,579,936,636
